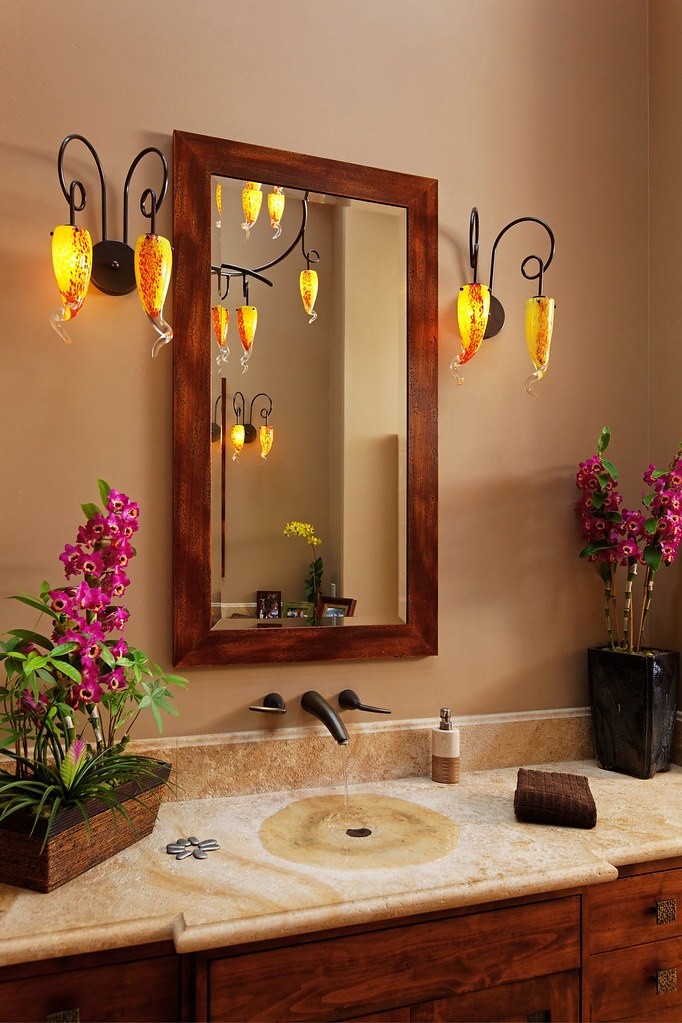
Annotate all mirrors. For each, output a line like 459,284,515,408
210,378,227,579
169,129,440,671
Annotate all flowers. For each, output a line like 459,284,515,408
0,478,142,787
572,426,682,652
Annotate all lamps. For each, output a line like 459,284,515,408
48,134,174,357
209,176,320,376
231,391,274,466
449,207,556,399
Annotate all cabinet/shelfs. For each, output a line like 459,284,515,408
0,855,682,1023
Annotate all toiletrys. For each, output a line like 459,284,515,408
430,706,462,786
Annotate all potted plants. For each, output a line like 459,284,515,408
0,581,188,893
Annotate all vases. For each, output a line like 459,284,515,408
588,642,682,780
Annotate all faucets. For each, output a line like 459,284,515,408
300,689,350,746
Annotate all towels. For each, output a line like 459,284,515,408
513,768,598,832
